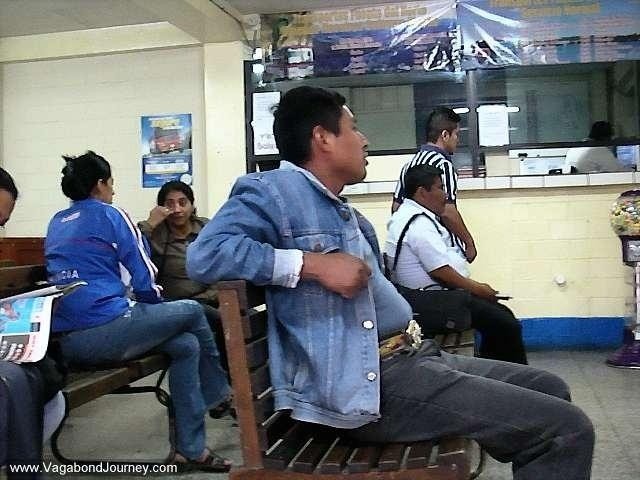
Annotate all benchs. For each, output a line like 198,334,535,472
216,280,486,480
0,237,176,474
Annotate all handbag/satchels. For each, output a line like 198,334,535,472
392,282,472,336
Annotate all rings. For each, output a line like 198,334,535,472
165,209,169,212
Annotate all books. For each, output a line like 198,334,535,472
0,281,89,361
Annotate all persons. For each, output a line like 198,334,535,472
186,86,596,480
383,164,528,368
137,180,239,420
44,150,236,473
394,107,477,263
1,166,48,480
565,121,636,172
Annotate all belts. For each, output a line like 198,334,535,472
378,319,423,356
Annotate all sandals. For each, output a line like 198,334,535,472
209,395,236,420
169,446,234,473
